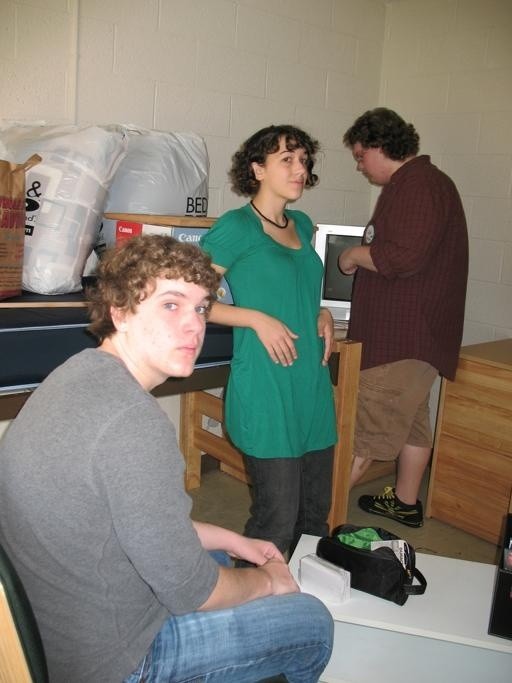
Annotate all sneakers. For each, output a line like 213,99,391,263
359,487,424,529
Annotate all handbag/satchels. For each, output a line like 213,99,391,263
299,553,352,607
0,153,44,302
315,523,427,606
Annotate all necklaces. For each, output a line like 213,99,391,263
250,199,288,229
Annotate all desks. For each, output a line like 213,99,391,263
0,286,362,542
248,533,512,683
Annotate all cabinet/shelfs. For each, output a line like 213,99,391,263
423,336,511,545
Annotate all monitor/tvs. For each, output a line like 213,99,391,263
314,223,367,321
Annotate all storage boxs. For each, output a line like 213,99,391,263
97,211,236,309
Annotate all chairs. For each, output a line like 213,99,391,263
1,543,51,682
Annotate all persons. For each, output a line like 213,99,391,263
1,232,334,683
338,107,470,529
198,125,339,569
